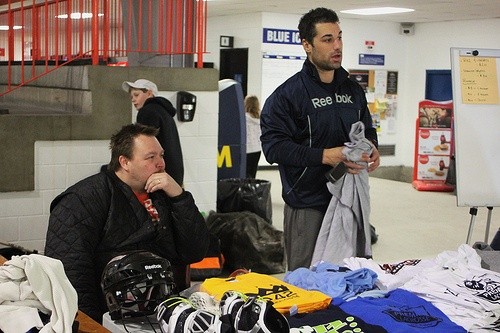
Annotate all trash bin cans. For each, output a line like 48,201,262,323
219,178,273,224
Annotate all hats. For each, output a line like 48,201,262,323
122,79,158,97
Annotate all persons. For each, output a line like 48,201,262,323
122,79,184,187
244,95,262,179
44,122,210,326
259,8,380,274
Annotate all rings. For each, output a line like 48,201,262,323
158,178,161,184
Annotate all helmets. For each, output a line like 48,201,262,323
100,250,176,320
